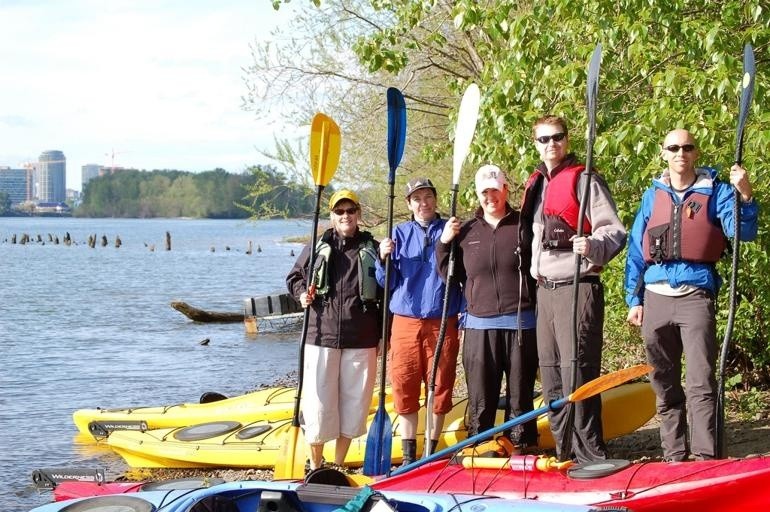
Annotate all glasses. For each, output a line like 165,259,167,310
662,145,697,152
535,133,566,143
334,208,357,215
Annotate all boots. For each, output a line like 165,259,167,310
420,438,438,459
398,439,416,468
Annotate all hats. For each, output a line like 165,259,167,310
405,177,435,198
329,190,359,210
475,165,507,193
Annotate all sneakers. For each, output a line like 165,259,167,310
333,463,355,475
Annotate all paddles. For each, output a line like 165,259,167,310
715,45,754,460
425,82,480,458
558,44,602,463
364,87,406,477
377,364,654,481
273,114,341,480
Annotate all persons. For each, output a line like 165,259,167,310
375,178,466,470
436,163,539,457
286,188,395,471
513,116,627,464
623,128,758,461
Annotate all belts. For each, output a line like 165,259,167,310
543,276,599,290
